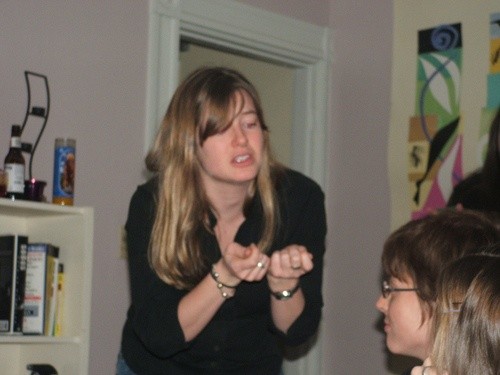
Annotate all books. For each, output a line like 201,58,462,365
0,235,65,337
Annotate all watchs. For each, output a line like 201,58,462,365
268,281,301,301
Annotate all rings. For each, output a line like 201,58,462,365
422,366,433,375
257,262,265,269
292,266,302,271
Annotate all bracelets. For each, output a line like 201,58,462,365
209,265,237,299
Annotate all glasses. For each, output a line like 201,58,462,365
381,279,418,298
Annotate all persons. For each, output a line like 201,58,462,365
117,67,328,375
376,105,500,375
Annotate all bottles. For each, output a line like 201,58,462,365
3,123,26,200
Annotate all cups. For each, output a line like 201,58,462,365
52,137,76,205
24,178,47,201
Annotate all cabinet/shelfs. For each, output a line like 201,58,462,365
0,195,94,375
